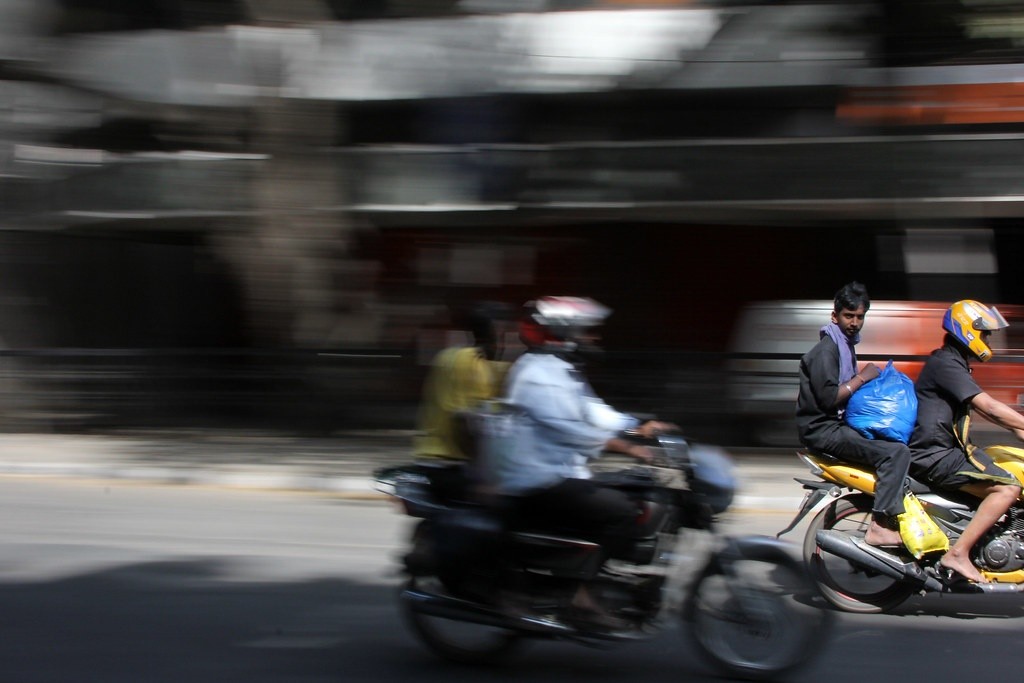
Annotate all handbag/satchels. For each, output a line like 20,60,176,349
845,359,917,444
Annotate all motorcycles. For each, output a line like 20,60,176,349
774,439,1024,615
370,428,834,683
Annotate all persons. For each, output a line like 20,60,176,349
405,283,680,627
792,281,910,546
906,299,1024,584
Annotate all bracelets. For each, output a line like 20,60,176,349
842,382,854,397
856,374,866,384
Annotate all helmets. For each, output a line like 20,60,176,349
519,296,613,358
942,299,1010,362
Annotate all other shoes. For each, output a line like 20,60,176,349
561,602,637,632
